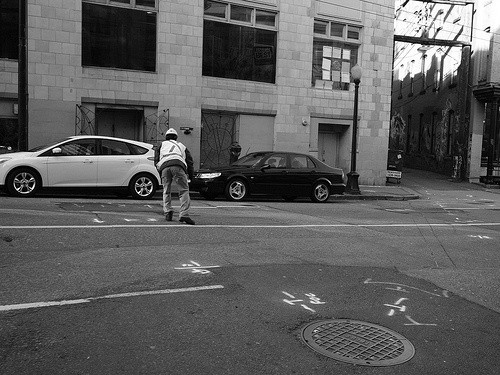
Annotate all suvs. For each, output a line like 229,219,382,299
0,135,164,200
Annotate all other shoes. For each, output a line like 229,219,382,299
166,211,173,220
179,216,195,225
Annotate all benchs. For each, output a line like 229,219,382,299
103,146,122,154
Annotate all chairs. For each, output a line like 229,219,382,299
292,161,300,169
267,159,277,167
277,160,285,168
77,145,88,155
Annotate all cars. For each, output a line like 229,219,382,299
187,150,346,202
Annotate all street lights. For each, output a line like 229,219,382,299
344,64,362,195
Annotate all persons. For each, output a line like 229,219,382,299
154,128,195,225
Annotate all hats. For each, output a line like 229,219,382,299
165,128,178,136
